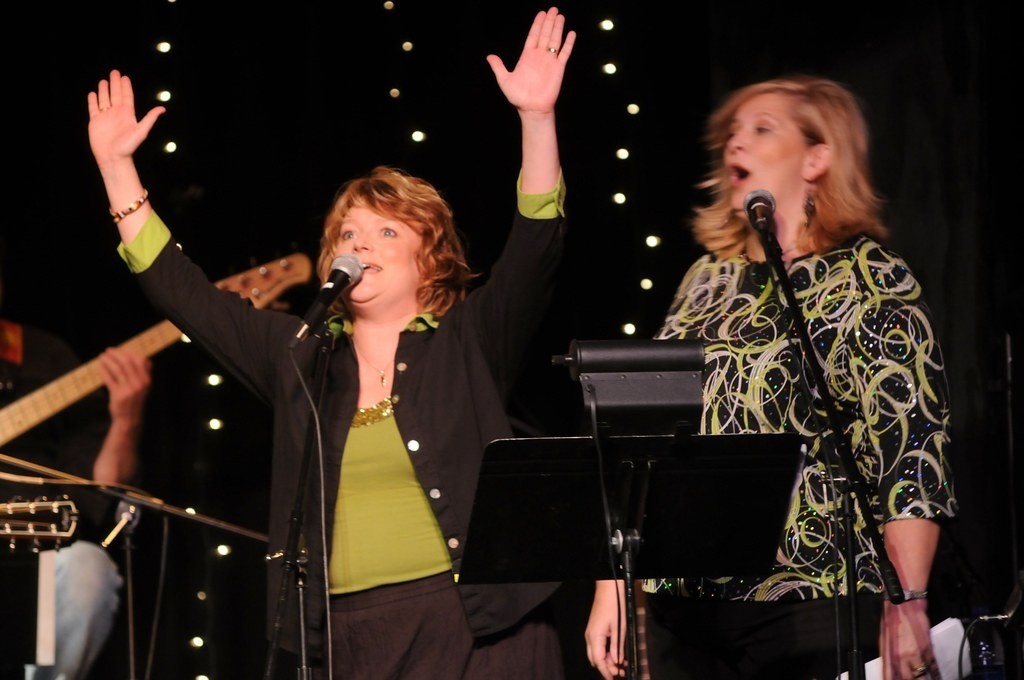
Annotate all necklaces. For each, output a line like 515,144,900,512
355,343,393,386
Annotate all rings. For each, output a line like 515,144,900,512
912,662,927,672
100,107,109,111
547,48,557,54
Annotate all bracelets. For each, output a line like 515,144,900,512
883,589,928,601
109,190,148,223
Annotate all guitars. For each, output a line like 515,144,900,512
0,253,312,448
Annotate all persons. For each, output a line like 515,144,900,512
0,318,151,680
585,79,959,680
87,6,575,680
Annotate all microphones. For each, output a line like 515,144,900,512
743,189,776,232
291,254,364,353
